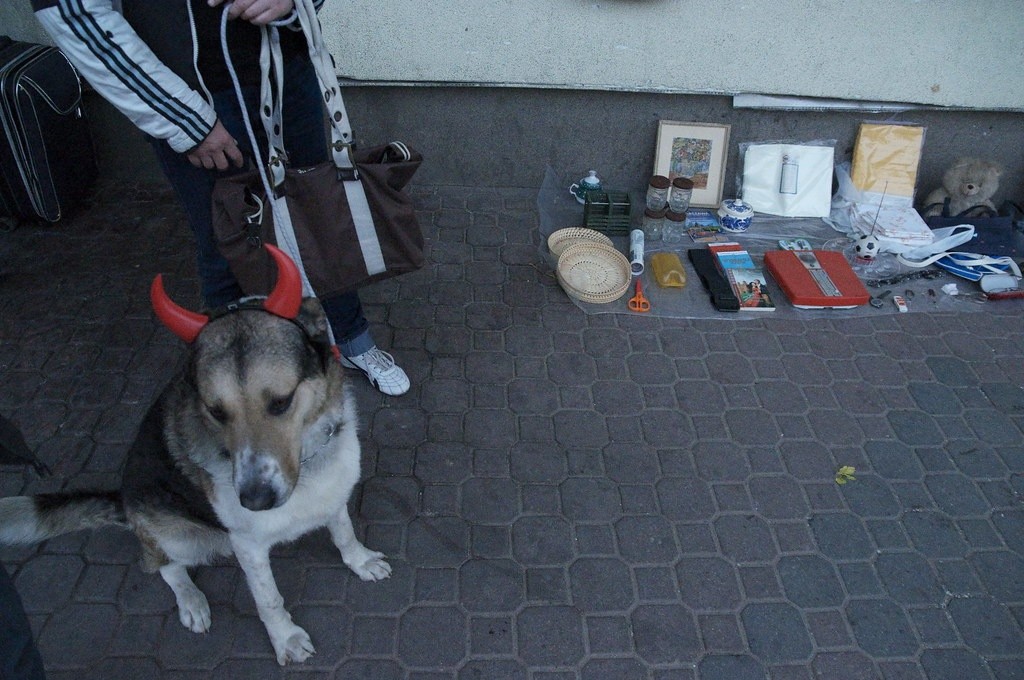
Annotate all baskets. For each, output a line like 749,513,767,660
547,226,633,303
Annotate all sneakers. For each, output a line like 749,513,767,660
335,341,410,394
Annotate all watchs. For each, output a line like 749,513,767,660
869,289,892,309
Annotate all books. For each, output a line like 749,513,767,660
714,249,756,281
722,266,777,312
683,208,729,244
706,241,743,278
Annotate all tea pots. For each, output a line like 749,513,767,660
569,170,604,206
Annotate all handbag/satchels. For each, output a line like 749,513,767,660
210,1,423,302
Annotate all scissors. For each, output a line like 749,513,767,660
627,278,650,312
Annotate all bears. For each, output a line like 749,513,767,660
923,153,1003,225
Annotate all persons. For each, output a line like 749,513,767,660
30,0,412,397
753,279,775,307
744,282,761,307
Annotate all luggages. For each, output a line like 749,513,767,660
0,36,104,227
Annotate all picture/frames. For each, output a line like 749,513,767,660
654,118,731,208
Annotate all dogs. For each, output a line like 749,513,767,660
0,294,396,666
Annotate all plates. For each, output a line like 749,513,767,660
822,237,902,282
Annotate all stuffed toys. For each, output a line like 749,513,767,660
921,154,1007,225
